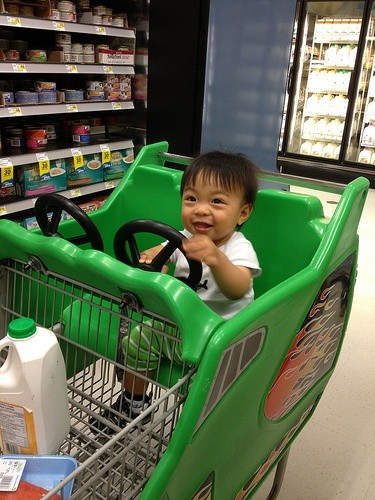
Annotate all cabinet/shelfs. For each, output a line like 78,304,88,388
0,0,147,235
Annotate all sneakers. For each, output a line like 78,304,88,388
88,391,154,438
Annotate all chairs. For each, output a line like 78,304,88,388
30,159,324,398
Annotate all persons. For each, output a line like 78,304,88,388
86,150,263,430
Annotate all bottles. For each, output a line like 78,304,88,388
0,317,70,458
